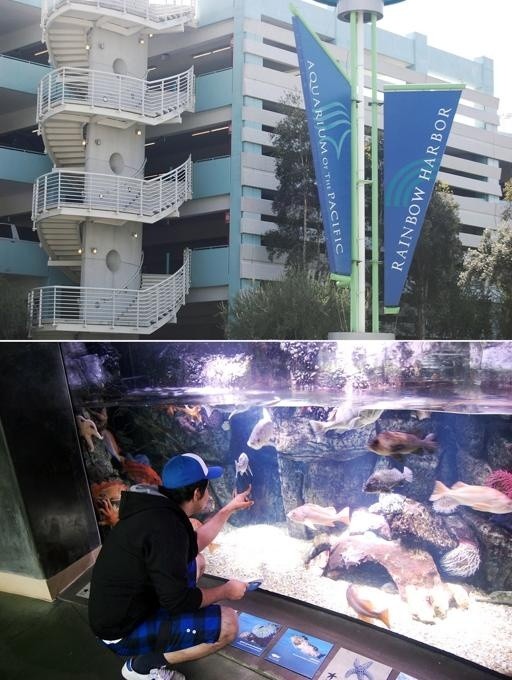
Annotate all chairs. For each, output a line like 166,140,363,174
120,658,186,680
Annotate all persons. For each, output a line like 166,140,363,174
88,453,254,680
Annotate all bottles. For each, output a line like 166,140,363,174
161,453,223,487
